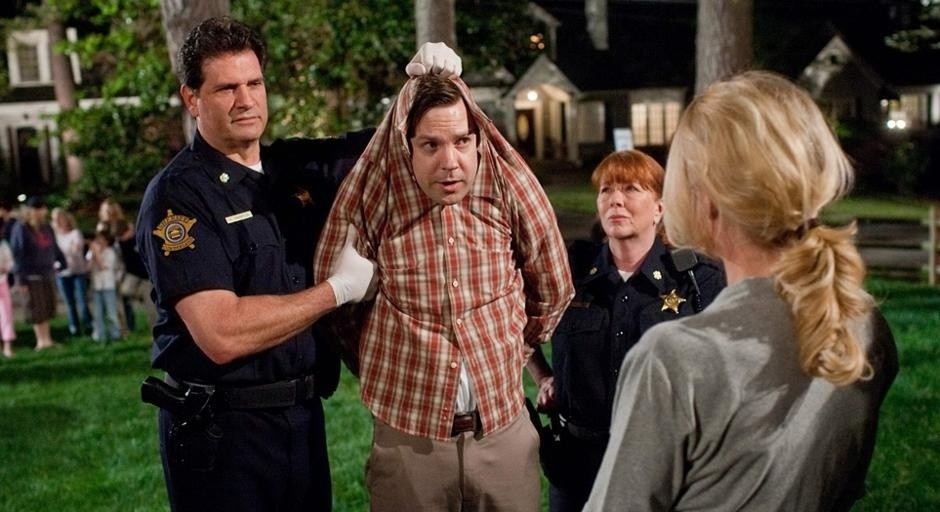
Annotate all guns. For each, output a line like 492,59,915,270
141,377,216,467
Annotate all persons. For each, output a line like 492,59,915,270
581,65,898,510
1,190,145,360
135,12,463,512
519,144,731,512
311,68,580,512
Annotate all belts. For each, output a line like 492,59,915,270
453,415,481,431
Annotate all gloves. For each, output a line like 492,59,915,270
326,222,380,308
405,41,463,78
